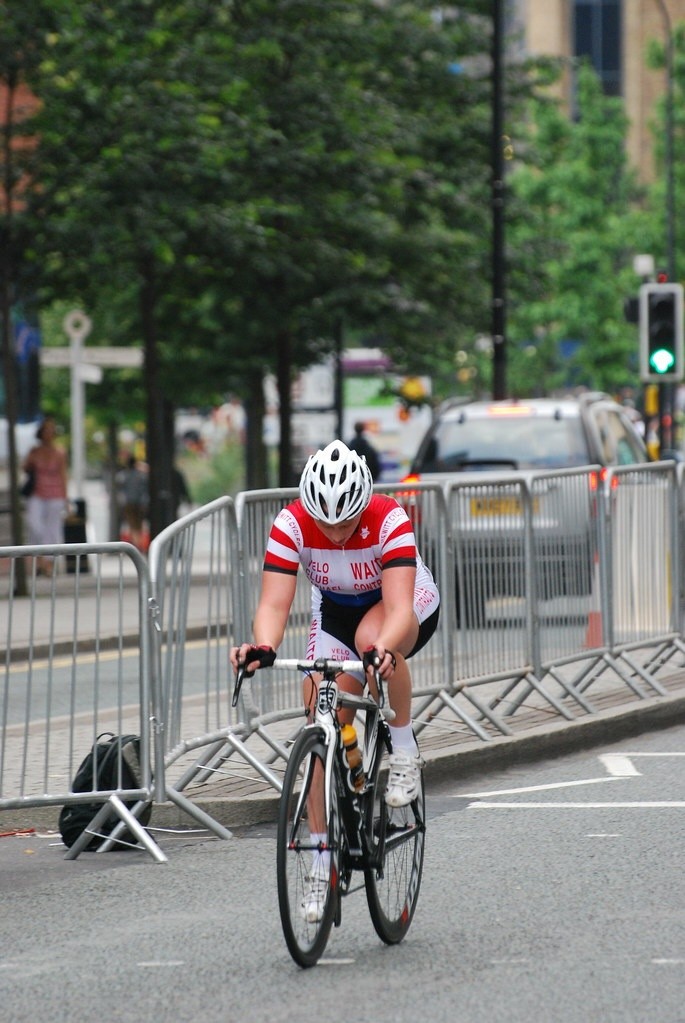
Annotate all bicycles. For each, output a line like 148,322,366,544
230,648,428,969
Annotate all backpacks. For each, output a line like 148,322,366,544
59,732,157,851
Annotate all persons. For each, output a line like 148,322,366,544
347,423,382,483
22,418,70,577
116,451,193,558
229,440,440,918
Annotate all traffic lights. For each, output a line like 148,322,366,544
639,282,685,384
624,298,641,325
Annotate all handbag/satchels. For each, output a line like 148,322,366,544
20,468,36,496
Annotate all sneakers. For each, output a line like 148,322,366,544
299,869,342,922
384,729,427,808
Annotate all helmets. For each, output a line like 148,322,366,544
298,439,372,525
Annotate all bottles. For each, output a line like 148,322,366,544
339,724,366,793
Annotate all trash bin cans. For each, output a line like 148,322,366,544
63,499,89,574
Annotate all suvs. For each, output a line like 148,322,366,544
394,390,654,629
